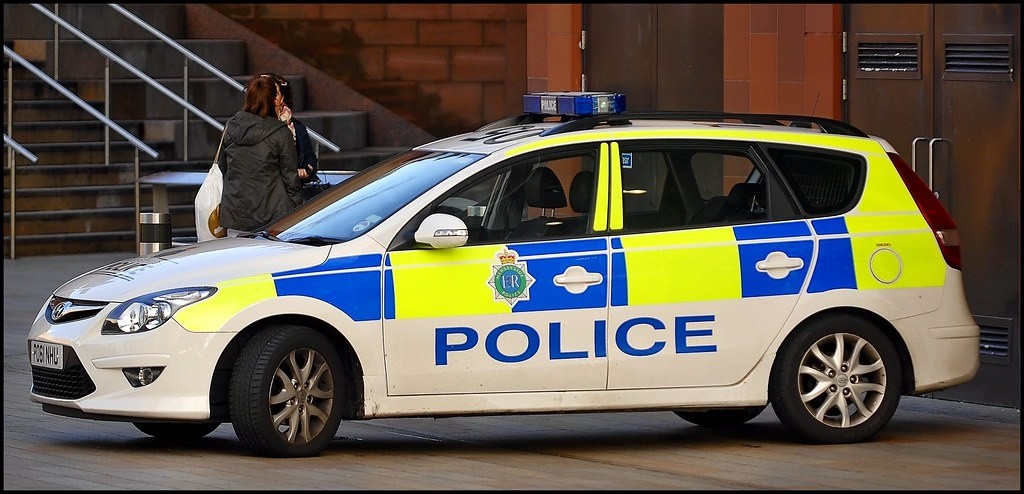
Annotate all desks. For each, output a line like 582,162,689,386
131,172,351,214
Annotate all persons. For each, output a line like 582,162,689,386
265,72,319,186
219,71,299,240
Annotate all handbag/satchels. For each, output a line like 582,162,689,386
300,168,330,199
194,121,227,242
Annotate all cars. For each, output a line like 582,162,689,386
24,89,981,459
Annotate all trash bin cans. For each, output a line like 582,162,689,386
139,213,173,257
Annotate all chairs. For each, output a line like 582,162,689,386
688,180,765,225
502,164,569,241
566,166,597,235
